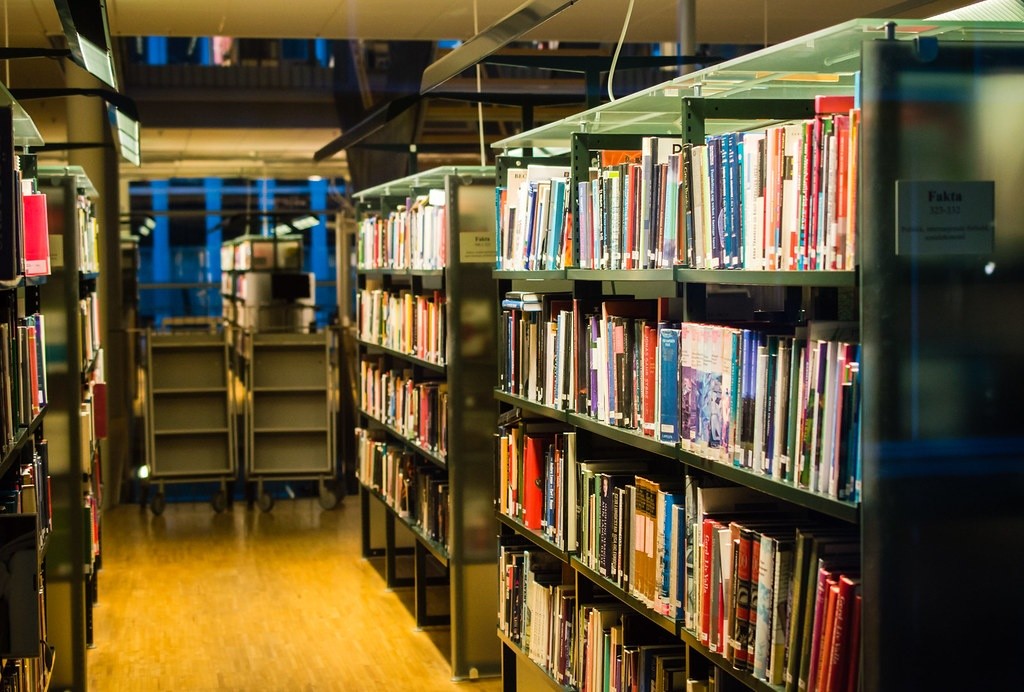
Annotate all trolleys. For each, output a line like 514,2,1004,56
244,325,346,514
137,327,241,517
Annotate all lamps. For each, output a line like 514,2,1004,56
420,0,585,94
8,88,142,168
312,92,521,161
292,213,321,230
270,224,293,236
0,33,119,90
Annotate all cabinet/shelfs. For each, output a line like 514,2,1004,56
137,319,239,514
491,17,1024,692
0,36,119,692
219,234,303,331
353,166,502,680
246,326,342,510
